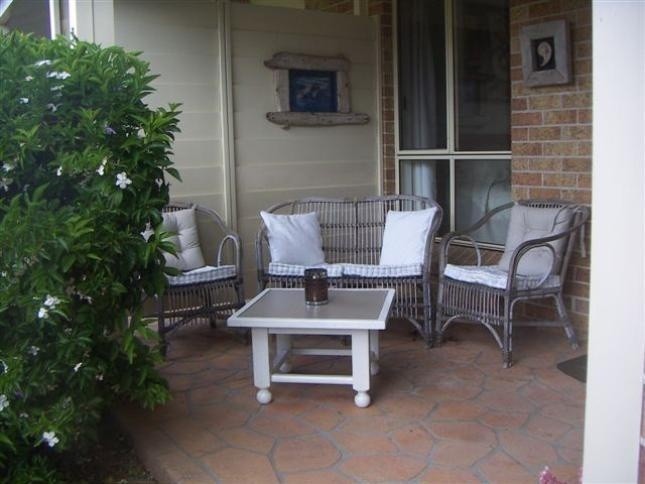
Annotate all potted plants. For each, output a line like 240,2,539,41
518,19,572,89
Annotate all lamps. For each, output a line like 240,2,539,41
146,203,247,356
255,196,442,348
436,198,590,368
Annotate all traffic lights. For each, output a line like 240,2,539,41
497,200,574,278
155,206,208,272
259,209,326,264
380,207,436,262
